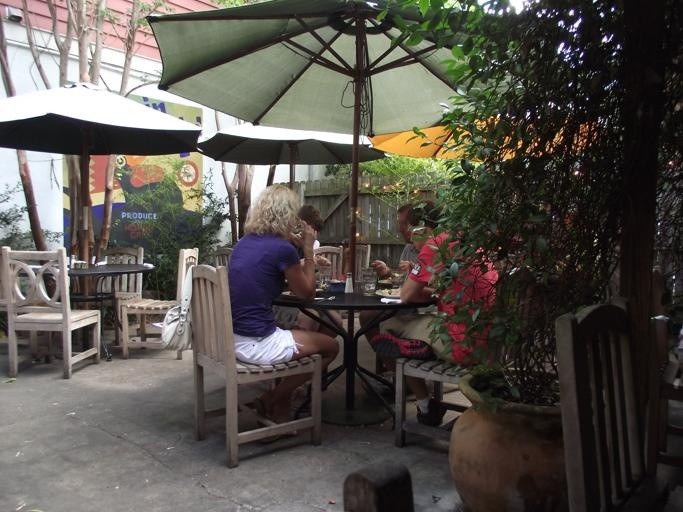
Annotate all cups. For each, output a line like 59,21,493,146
318,265,331,292
360,268,377,296
391,271,405,287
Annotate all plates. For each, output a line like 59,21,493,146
377,280,391,284
375,289,401,298
282,288,323,298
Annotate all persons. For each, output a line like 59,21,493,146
272,205,343,338
227,184,340,443
359,203,420,395
370,200,499,427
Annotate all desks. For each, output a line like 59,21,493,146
18,263,154,362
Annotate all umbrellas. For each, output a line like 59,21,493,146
370,116,597,164
197,122,386,189
144,0,571,410
0,83,202,353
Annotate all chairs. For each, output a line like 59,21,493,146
313,241,370,283
79,247,145,344
120,248,198,360
2,247,101,377
643,265,683,475
183,262,324,468
552,300,682,511
393,267,538,446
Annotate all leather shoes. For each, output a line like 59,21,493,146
254,391,289,442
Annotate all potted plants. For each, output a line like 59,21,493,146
374,0,618,511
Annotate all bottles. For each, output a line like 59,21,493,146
344,273,354,293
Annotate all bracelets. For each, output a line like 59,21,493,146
304,257,313,262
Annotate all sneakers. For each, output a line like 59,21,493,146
417,398,449,427
362,369,395,394
